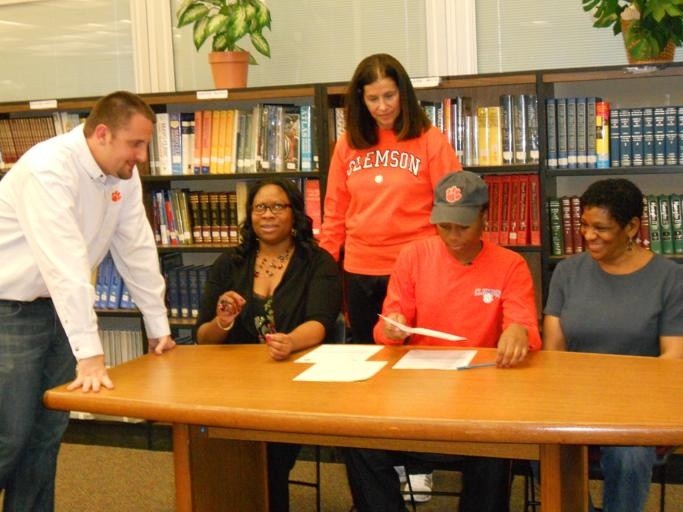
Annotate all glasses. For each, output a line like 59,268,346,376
252,200,292,214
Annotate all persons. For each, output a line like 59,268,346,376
344,170,542,511
191,177,342,512
317,53,465,503
529,179,683,511
0,92,176,511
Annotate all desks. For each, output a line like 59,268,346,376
39,333,682,512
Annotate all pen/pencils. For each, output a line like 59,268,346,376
457,363,496,369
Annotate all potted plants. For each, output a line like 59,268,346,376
171,0,277,88
578,1,683,67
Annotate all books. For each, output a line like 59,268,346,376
85,180,325,365
146,104,351,177
1,108,87,177
415,92,683,261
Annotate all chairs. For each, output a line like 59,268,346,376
285,312,347,511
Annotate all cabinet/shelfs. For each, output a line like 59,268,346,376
139,83,333,465
318,68,543,332
538,58,683,326
0,95,150,454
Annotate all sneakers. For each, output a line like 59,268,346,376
402,472,432,502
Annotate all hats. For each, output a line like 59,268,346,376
429,171,489,228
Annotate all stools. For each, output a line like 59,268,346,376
525,462,670,510
391,450,528,511
